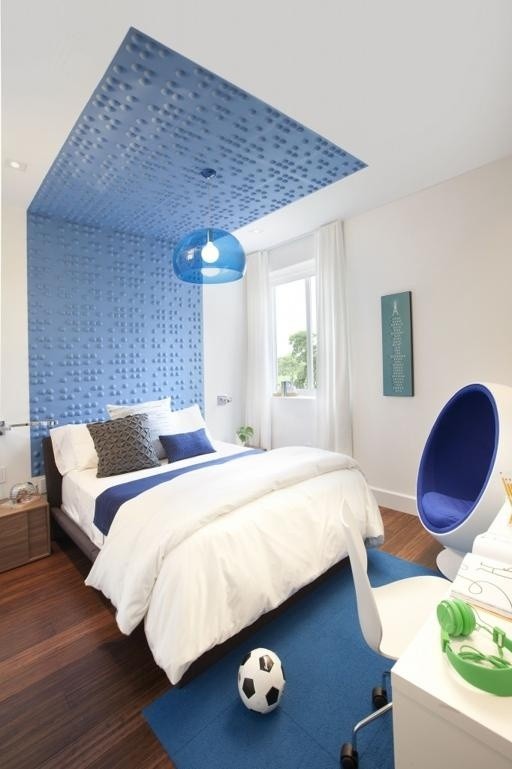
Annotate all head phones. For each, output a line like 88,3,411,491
437,595,512,697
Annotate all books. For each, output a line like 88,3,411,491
446,487,512,621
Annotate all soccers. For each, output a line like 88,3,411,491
237,648,286,713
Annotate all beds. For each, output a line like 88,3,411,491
39,395,384,685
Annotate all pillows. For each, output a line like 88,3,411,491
49,396,218,479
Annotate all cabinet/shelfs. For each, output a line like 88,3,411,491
387,510,512,766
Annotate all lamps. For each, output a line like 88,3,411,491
174,166,247,283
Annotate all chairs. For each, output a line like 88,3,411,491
412,379,512,582
335,506,463,769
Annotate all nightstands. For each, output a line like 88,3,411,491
0,490,56,573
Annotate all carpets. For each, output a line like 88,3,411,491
137,539,448,769
